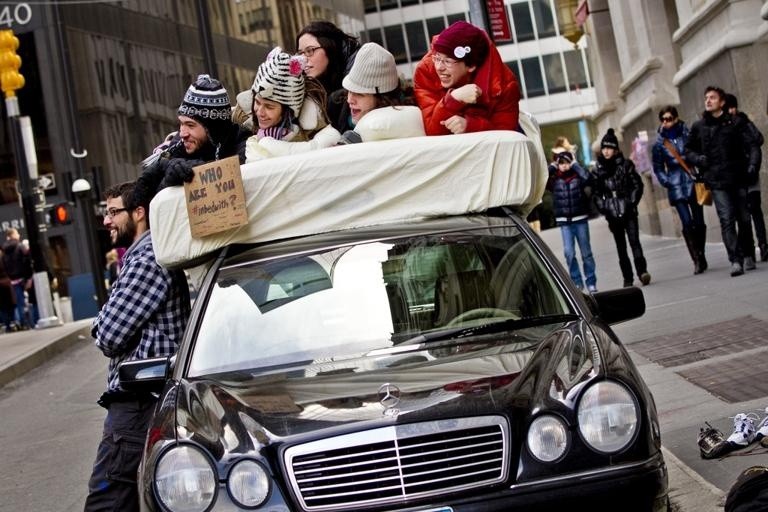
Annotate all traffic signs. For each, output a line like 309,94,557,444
28,172,57,195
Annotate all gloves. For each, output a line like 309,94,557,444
163,158,194,187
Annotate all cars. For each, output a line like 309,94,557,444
110,207,672,512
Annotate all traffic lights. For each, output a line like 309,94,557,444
53,203,76,227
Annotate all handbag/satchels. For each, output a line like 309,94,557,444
694,181,713,206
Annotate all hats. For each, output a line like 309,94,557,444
431,22,490,67
341,42,399,95
600,128,618,148
250,46,306,129
177,74,233,148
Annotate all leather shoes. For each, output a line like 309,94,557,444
730,261,744,275
743,257,755,270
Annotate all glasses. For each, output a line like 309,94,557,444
297,45,322,56
103,207,128,217
660,116,676,122
430,51,460,68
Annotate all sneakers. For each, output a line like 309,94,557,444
640,272,651,286
587,284,597,294
698,421,730,459
756,406,768,440
760,244,768,261
727,413,760,447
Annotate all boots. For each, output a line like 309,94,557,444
683,224,707,274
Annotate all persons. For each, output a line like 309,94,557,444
725,466,768,512
652,86,767,276
0,223,35,332
84,186,189,508
544,136,598,300
594,128,651,292
413,20,521,136
139,21,427,191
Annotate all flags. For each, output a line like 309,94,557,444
572,2,590,26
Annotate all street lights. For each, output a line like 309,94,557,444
0,26,61,329
70,178,110,307
